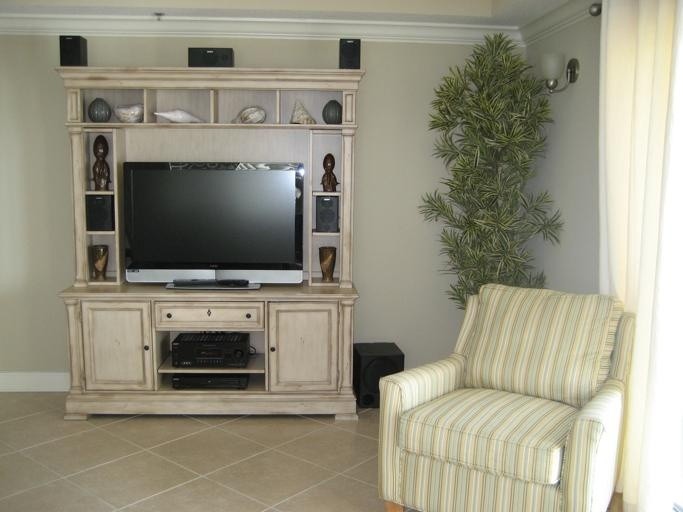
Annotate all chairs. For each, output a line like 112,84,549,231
378,293,636,512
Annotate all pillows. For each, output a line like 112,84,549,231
464,284,623,409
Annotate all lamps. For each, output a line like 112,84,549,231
541,52,579,94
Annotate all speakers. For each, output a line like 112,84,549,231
59,35,87,65
85,194,115,231
187,47,236,67
316,195,339,234
353,341,405,409
339,38,361,69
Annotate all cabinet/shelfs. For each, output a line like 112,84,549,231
55,66,367,421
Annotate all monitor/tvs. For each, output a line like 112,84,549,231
122,159,304,290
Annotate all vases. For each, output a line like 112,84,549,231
319,247,336,283
92,245,108,281
88,98,111,122
322,100,342,124
